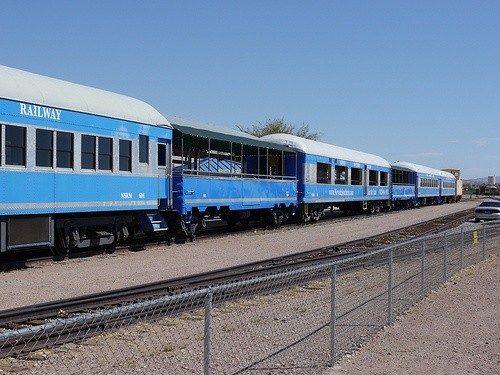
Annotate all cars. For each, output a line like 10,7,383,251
473,200,500,223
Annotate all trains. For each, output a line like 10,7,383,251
1,64,463,266
